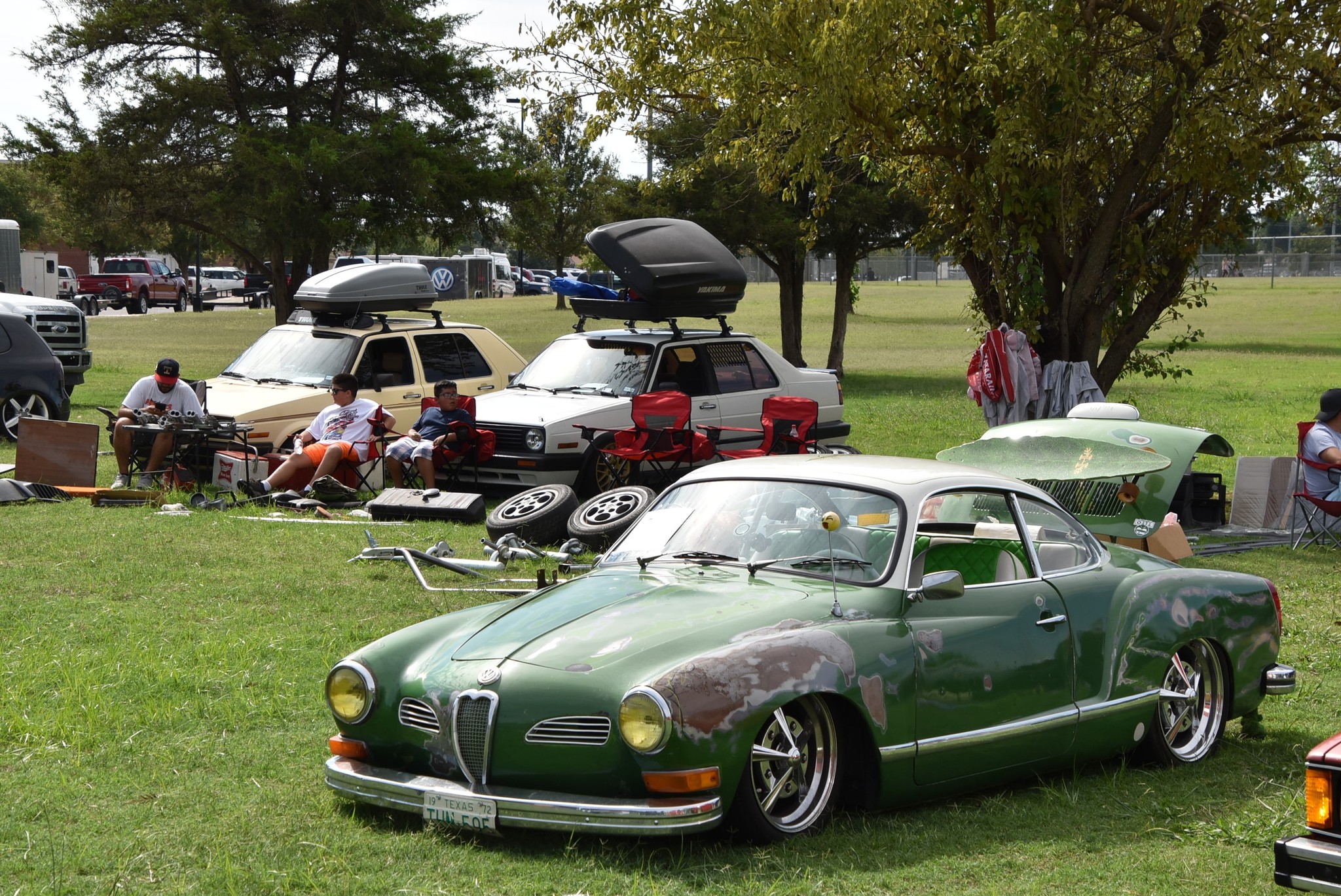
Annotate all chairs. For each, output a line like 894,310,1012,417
367,394,496,491
1288,421,1341,553
696,396,821,461
287,403,390,491
97,378,214,491
570,388,716,492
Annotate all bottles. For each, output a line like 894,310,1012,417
294,438,304,454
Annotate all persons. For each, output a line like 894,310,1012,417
386,379,473,488
111,357,205,492
238,373,398,504
1301,387,1341,502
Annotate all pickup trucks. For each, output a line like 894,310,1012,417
76,257,189,313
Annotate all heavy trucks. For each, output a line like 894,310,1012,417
0,220,93,399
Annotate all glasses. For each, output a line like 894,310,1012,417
436,394,460,400
332,388,348,394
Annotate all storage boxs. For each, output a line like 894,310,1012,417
566,217,748,320
262,453,315,494
370,487,486,523
212,450,269,491
291,259,439,313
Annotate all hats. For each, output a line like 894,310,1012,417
154,358,179,385
1313,388,1341,421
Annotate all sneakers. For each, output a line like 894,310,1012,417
237,477,269,498
135,473,153,490
297,488,310,498
110,472,130,490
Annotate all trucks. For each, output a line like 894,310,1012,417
332,247,516,301
20,248,59,301
188,261,312,312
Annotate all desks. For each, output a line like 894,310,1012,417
118,418,255,502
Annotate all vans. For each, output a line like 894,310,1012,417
199,266,247,294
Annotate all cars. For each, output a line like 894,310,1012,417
176,263,528,488
176,266,211,296
510,266,625,296
0,312,74,443
325,401,1298,851
418,218,850,490
58,265,79,294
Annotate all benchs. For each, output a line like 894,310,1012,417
766,511,1085,599
